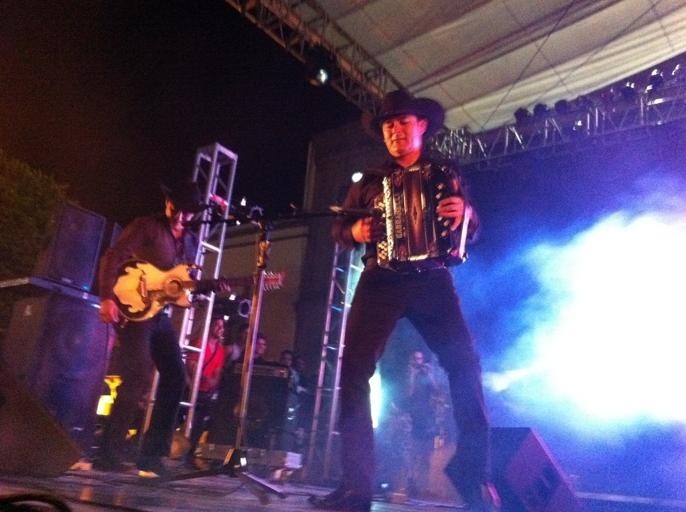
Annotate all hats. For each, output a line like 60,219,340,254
160,182,221,213
368,89,445,140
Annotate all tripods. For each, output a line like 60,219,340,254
139,230,285,505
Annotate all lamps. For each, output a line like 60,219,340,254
310,67,329,87
513,62,686,129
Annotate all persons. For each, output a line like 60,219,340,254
316,92,502,511
97,181,230,482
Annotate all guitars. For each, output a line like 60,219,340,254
110,259,282,323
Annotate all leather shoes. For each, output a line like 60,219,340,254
308,483,372,511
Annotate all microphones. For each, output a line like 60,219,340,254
338,207,383,219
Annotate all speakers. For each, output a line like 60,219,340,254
32,200,107,292
443,427,583,512
0,295,115,472
